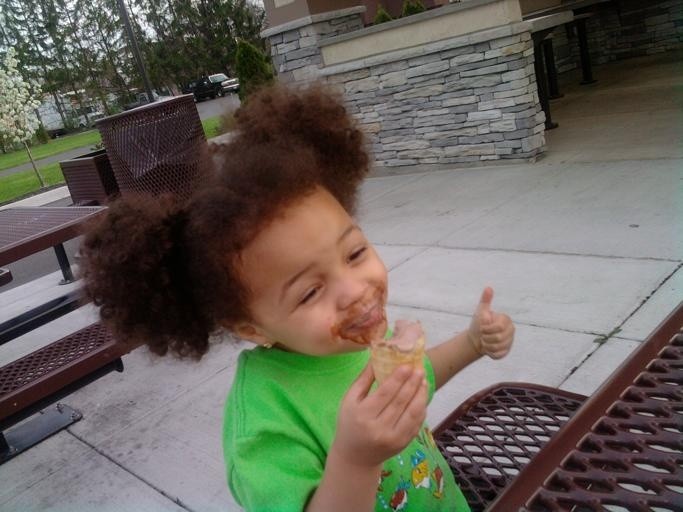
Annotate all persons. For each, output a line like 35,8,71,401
80,84,516,512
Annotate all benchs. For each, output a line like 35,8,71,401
434,381,636,510
0,315,144,466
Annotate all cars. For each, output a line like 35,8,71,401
190,73,239,103
122,89,157,110
71,106,104,129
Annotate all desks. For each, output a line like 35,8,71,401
0,205,111,465
481,297,682,512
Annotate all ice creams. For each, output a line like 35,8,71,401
368,321,423,391
